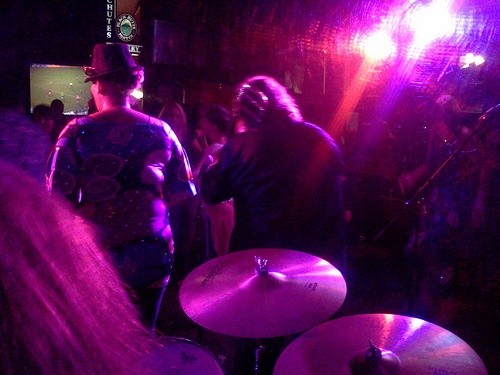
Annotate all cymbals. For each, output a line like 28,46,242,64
274,313,488,375
178,248,347,340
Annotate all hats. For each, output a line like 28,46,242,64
85,40,142,83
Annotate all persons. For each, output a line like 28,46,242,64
1,70,235,256
388,95,500,374
0,158,161,375
42,41,200,337
197,75,353,375
